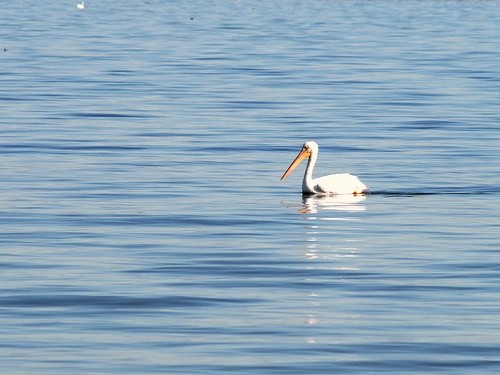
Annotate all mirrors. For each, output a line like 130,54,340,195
280,141,367,196
